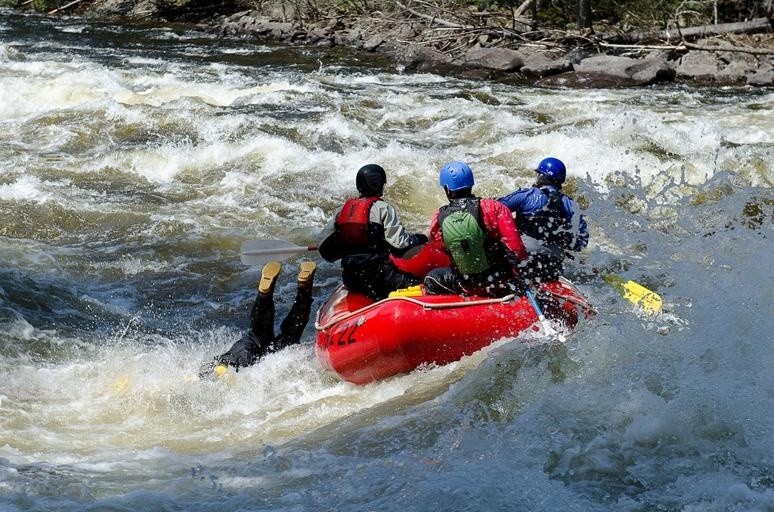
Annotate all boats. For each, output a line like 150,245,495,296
313,276,577,385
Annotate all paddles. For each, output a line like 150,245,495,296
241,239,319,267
526,288,566,343
565,252,663,315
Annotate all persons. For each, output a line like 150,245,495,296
201,260,317,381
316,164,430,301
382,160,527,297
484,158,589,287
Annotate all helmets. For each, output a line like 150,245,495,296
533,157,566,184
440,160,474,192
356,163,387,197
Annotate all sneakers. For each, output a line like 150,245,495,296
258,260,281,294
297,260,317,287
